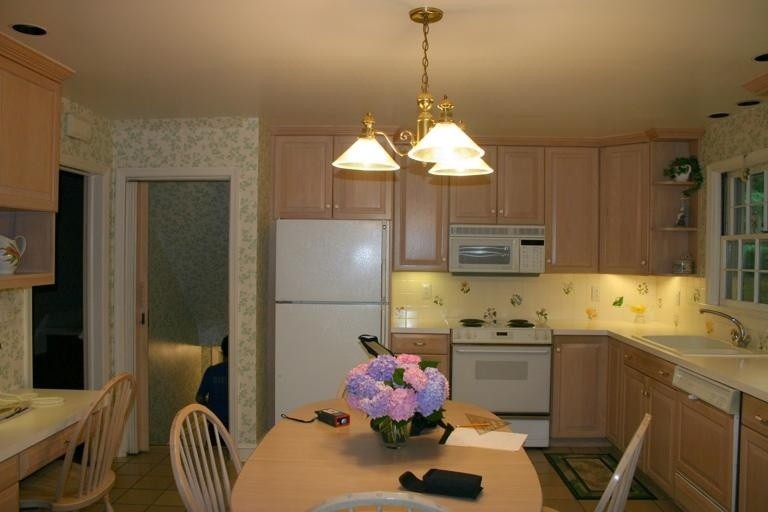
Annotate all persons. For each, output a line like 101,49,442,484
196,335,228,447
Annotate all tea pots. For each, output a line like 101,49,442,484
0,234,27,274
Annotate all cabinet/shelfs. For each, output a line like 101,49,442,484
395,139,449,273
448,144,544,224
391,333,451,398
736,391,767,512
599,142,705,278
608,337,623,452
1,32,76,291
623,344,675,499
543,146,599,275
269,127,396,221
552,333,609,439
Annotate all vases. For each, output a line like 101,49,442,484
378,419,410,453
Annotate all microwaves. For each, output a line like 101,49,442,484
448,224,546,275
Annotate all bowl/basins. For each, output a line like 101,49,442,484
672,259,694,274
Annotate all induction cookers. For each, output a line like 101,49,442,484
454,317,548,330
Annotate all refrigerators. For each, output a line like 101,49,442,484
273,218,390,425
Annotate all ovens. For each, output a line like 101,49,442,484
450,347,552,448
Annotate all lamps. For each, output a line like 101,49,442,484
331,9,495,178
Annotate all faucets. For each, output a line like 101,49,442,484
699,308,751,348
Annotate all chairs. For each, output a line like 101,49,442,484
168,404,242,511
308,492,449,512
20,372,138,512
540,412,652,511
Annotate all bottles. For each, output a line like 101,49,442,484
674,192,691,227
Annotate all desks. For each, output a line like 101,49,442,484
230,398,544,512
1,387,115,511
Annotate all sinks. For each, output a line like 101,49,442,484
632,331,751,356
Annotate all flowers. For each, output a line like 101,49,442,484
341,354,450,442
